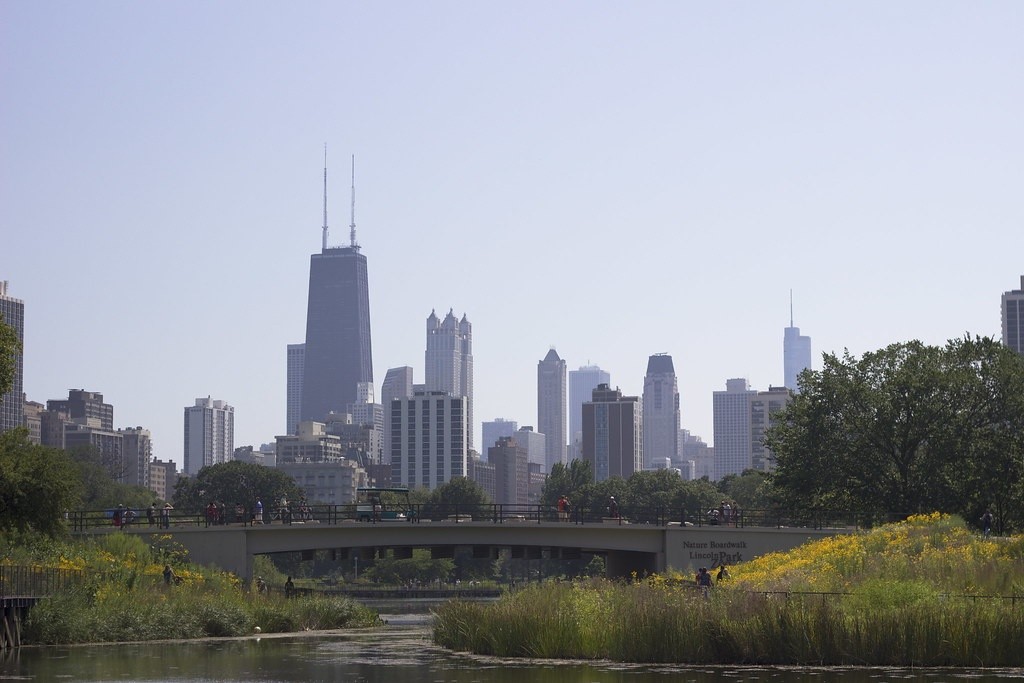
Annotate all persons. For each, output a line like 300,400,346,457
207,502,217,527
161,503,173,529
456,578,460,586
255,497,264,526
146,503,157,528
219,499,227,527
123,507,136,523
697,563,728,598
162,565,175,586
269,490,310,524
721,502,739,528
235,503,245,523
557,495,573,522
707,507,720,525
284,576,294,599
607,496,619,518
113,504,126,530
980,509,994,540
257,576,266,595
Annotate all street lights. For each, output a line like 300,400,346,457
354,556,358,578
